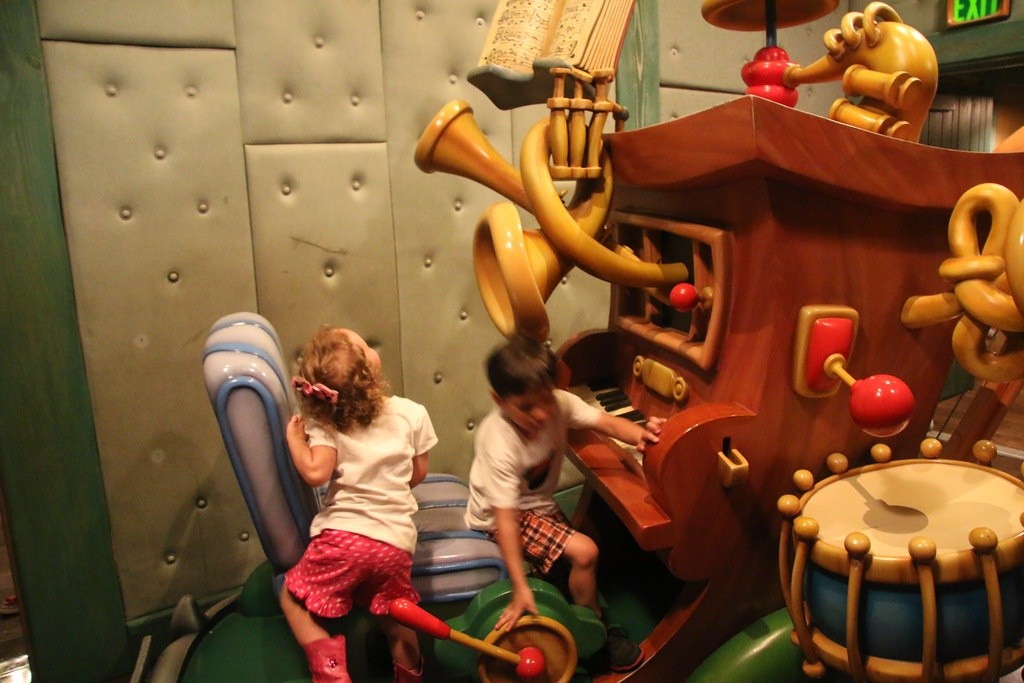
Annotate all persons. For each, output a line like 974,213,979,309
461,334,669,672
283,325,438,683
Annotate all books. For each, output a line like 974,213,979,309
478,0,637,82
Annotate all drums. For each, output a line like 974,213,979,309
776,437,1024,679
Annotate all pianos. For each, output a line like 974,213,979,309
549,100,1024,683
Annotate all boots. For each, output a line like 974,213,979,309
393,656,424,683
304,634,353,683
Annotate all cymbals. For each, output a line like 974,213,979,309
700,1,840,35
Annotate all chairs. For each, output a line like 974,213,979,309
202,312,507,605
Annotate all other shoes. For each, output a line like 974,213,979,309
589,635,643,671
